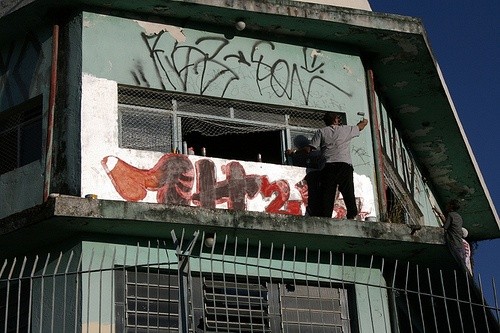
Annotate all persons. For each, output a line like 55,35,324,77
283,110,368,220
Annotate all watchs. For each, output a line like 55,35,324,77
293,148,296,157
